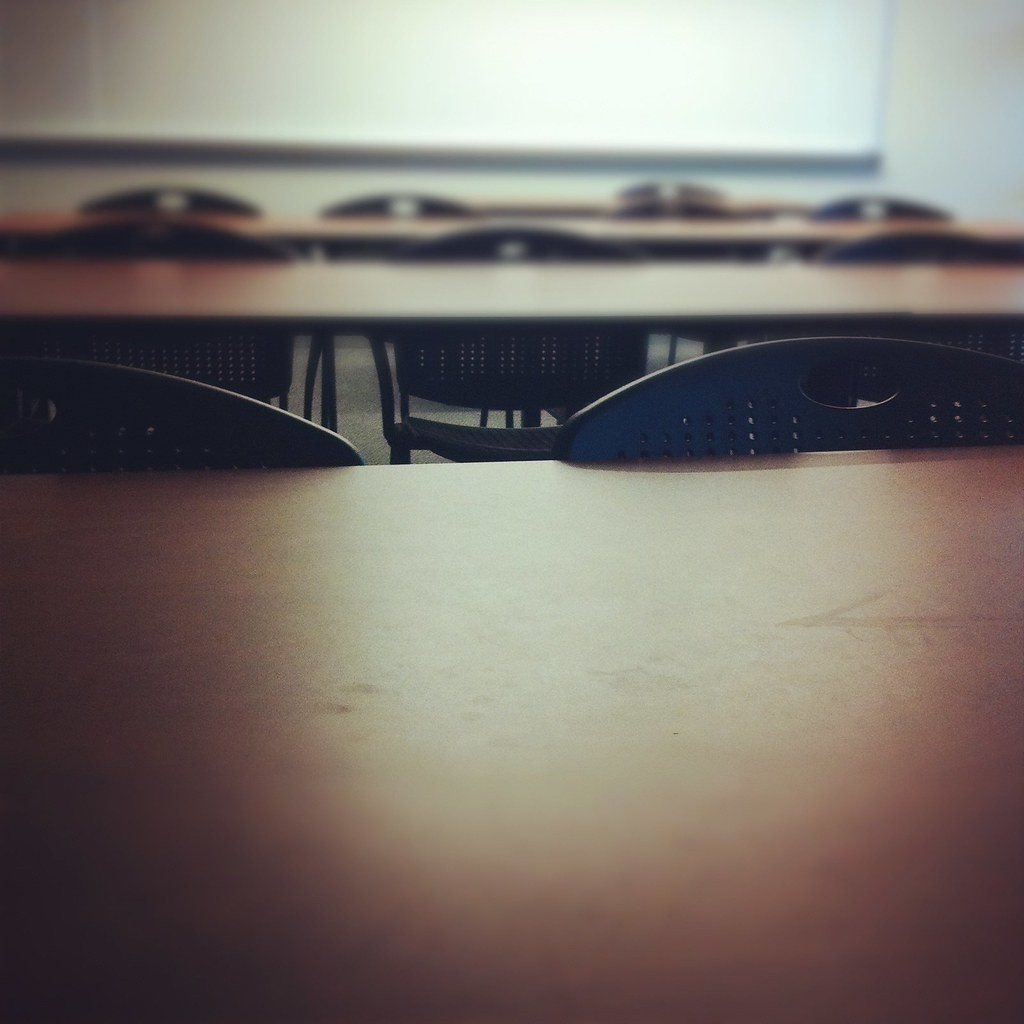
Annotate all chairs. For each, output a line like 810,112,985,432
0,179,1023,470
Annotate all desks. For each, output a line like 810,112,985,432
0,210,1023,240
0,441,1024,1024
0,251,1024,432
